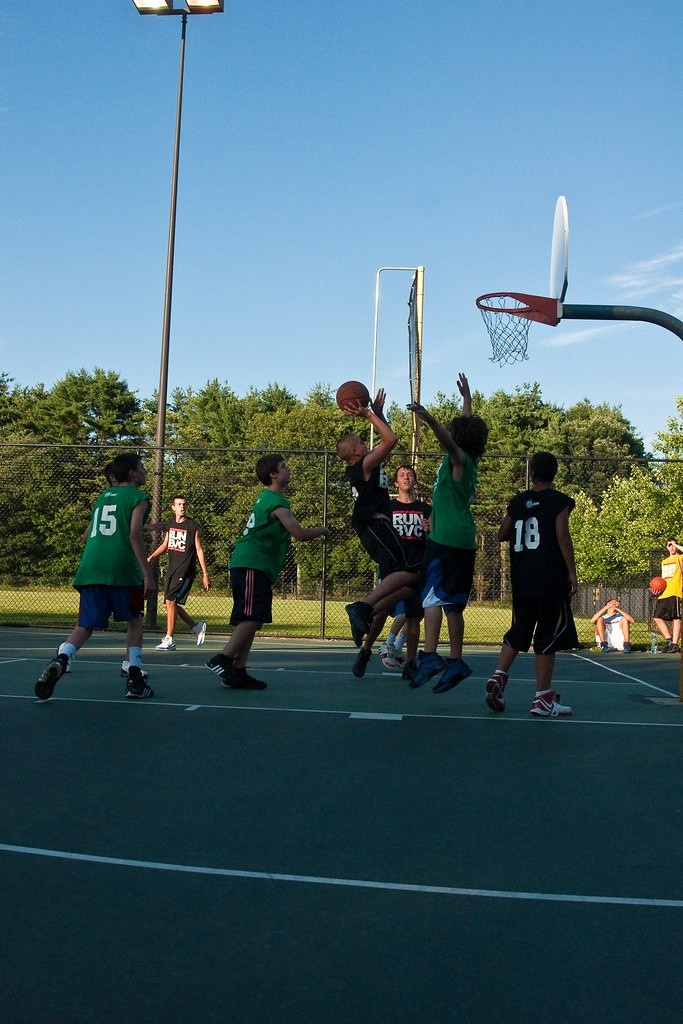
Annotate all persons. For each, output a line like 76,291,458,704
147,495,210,650
649,537,683,653
378,464,433,680
486,452,578,717
409,373,489,694
204,454,330,690
335,388,420,677
590,599,635,653
34,452,154,699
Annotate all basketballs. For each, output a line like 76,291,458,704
650,576,667,594
336,381,370,415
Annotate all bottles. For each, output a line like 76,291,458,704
652,633,658,653
590,647,601,652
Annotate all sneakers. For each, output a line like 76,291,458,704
410,649,445,689
155,636,176,651
485,674,505,712
392,648,408,671
120,661,148,679
125,680,154,698
600,641,609,653
191,622,206,647
57,642,73,670
205,655,229,678
623,642,631,653
352,647,372,677
221,671,268,690
35,658,69,700
432,657,473,694
530,690,572,717
402,659,417,679
661,640,673,653
350,618,363,648
666,642,680,653
379,641,396,669
345,600,375,634
